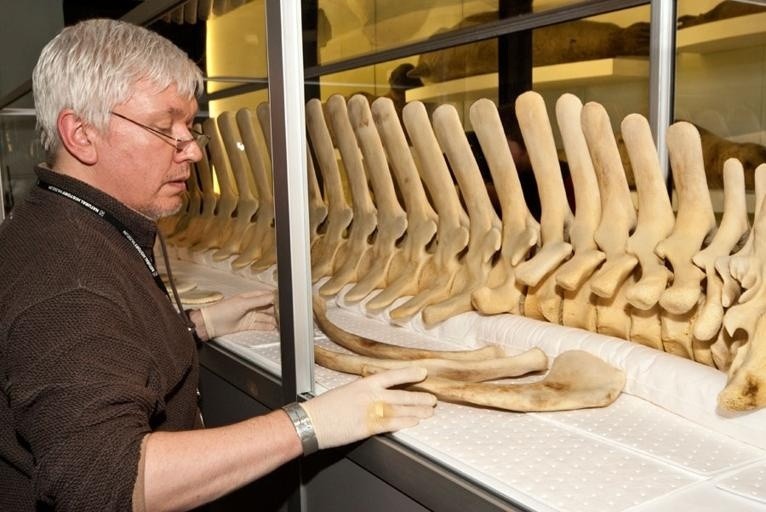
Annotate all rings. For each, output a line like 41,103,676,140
372,401,389,423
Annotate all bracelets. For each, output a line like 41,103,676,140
281,399,321,458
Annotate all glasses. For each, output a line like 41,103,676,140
108,110,212,158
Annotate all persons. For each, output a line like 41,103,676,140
0,19,440,511
